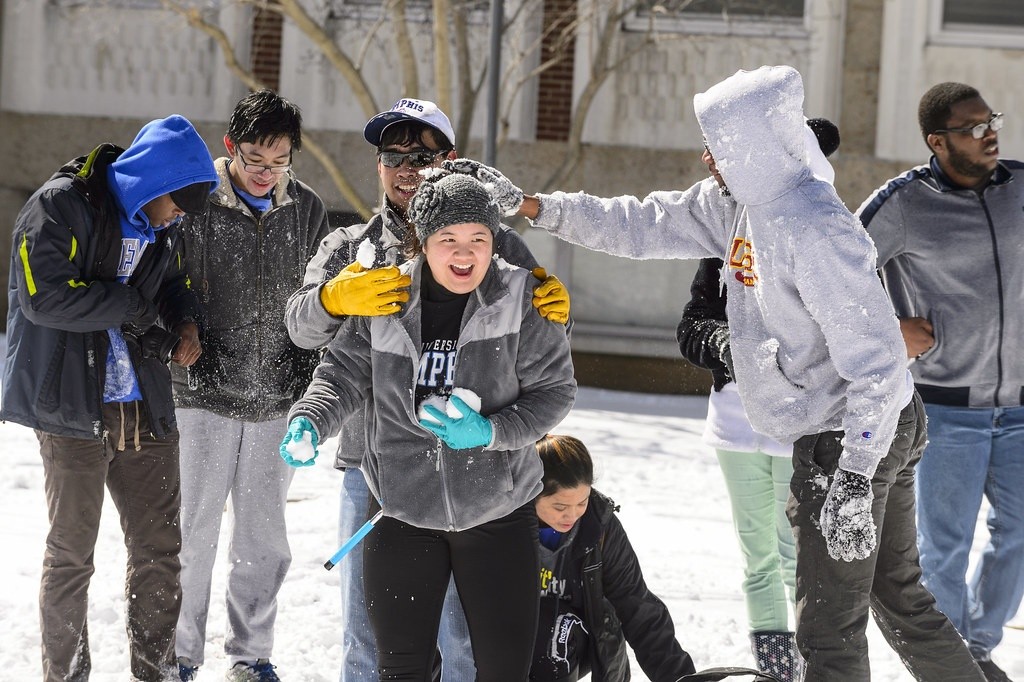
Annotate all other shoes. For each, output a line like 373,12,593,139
977,659,1014,682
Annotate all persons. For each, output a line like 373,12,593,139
1,114,221,682
676,118,840,682
851,80,1024,682
277,173,577,682
168,89,331,682
528,433,697,682
439,64,990,682
283,99,573,681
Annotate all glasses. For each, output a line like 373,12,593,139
378,149,447,168
935,112,1004,140
234,142,293,174
702,137,712,154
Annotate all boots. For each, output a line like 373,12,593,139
749,630,808,682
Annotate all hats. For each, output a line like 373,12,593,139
407,174,500,244
171,182,211,215
363,98,455,149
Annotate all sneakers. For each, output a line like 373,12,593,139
227,659,281,682
177,657,198,682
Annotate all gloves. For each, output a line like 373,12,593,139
275,337,320,403
121,293,182,366
708,327,736,383
441,158,522,217
820,468,875,561
279,418,319,467
320,260,412,316
186,330,235,398
419,395,492,449
531,265,569,325
